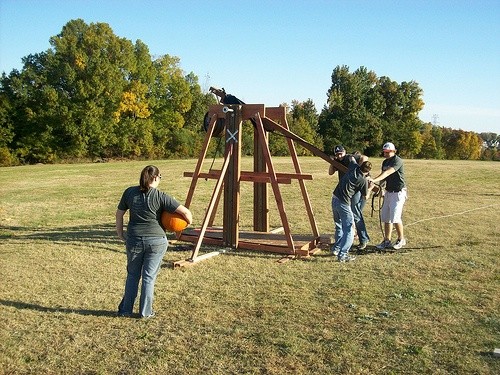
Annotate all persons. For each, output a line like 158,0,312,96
366,142,407,250
331,156,375,262
328,145,370,250
115,165,193,318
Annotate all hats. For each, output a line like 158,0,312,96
334,145,344,156
381,142,395,153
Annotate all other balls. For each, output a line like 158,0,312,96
161,208,189,232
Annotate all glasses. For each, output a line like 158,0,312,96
158,174,161,178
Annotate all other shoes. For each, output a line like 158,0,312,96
375,240,391,249
332,249,339,255
337,254,356,261
392,238,406,250
359,240,370,249
